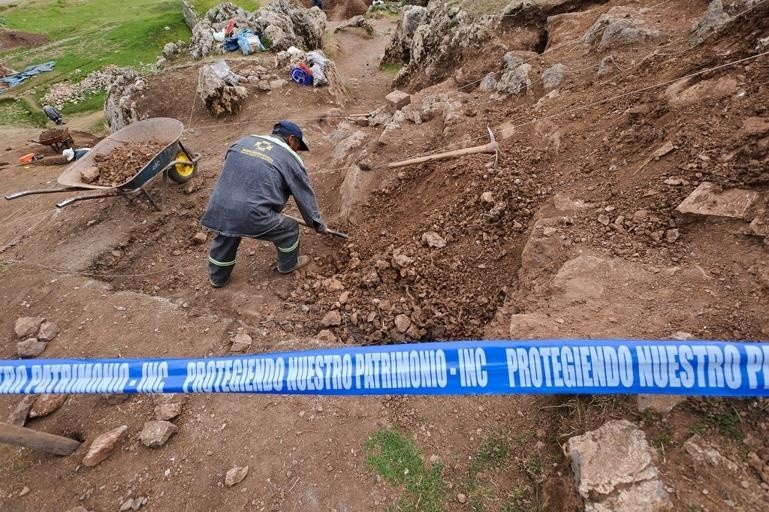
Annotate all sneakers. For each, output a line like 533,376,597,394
277,255,310,274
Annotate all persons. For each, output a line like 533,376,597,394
43,103,62,124
199,119,330,288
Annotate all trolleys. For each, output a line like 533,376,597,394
4,116,202,212
27,128,74,153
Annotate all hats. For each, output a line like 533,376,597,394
271,120,309,151
